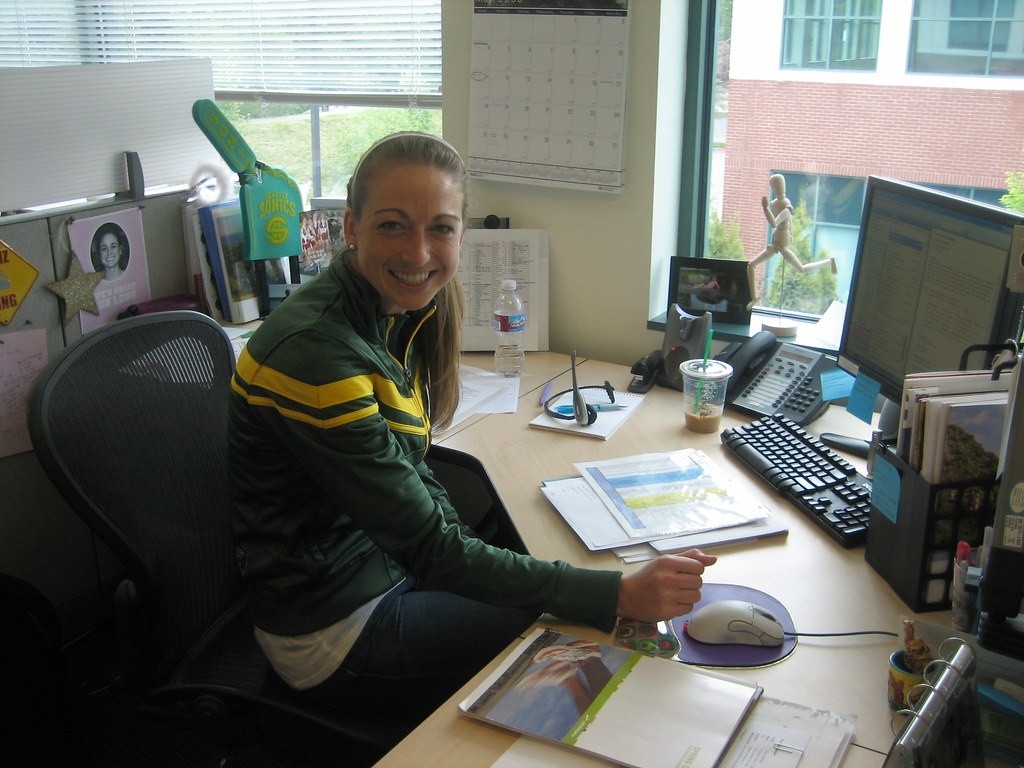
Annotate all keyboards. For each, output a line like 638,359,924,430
720,413,876,549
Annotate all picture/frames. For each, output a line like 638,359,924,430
667,256,752,325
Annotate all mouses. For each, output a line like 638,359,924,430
684,599,784,646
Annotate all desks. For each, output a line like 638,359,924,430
215,318,1012,768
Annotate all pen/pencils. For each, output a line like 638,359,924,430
553,403,628,415
955,540,985,634
538,382,551,406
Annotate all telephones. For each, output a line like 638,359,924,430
712,331,839,426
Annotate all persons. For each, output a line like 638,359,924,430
229,138,718,722
746,172,836,310
96,226,125,280
301,210,332,260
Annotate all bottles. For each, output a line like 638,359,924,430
492,279,525,377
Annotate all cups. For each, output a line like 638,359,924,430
680,359,732,434
888,650,938,714
952,546,986,631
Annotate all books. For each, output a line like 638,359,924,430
897,371,1014,546
457,625,765,768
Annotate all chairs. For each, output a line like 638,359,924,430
26,309,423,757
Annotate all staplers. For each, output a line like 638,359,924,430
627,349,662,393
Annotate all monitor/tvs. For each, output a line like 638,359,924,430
820,174,1024,459
